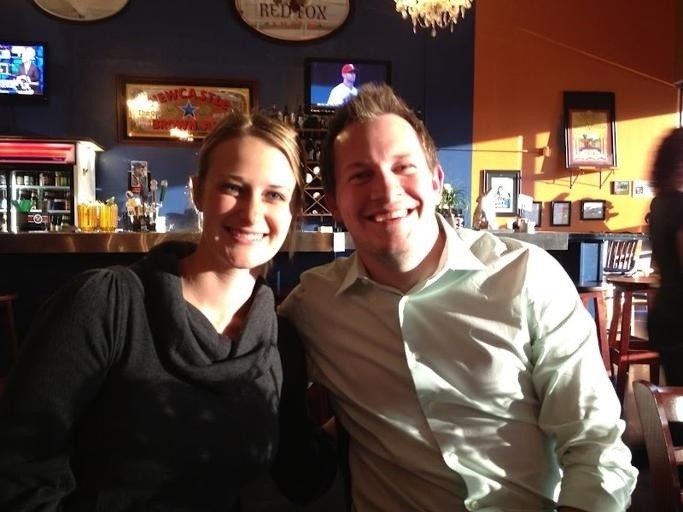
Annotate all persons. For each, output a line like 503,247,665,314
18,51,39,89
646,126,683,387
274,80,639,512
0,112,334,512
326,64,358,106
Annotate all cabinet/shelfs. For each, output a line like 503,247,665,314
289,125,333,217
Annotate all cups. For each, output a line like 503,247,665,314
520,221,535,233
76,203,98,232
97,203,118,232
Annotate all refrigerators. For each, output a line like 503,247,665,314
0,136,106,232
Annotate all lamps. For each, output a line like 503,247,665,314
393,0,474,39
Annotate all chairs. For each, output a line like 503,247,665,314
629,379,682,512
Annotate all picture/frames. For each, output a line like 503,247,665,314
112,73,261,149
480,167,521,217
518,201,542,227
549,201,571,227
561,90,617,171
612,180,628,195
579,199,606,221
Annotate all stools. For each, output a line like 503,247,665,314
606,275,662,413
576,286,611,372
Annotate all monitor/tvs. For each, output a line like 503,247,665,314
304,56,391,116
0,40,50,104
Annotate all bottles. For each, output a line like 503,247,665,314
17,188,55,210
49,213,72,231
63,191,70,209
0,174,7,231
16,170,70,185
271,103,305,129
302,136,322,159
